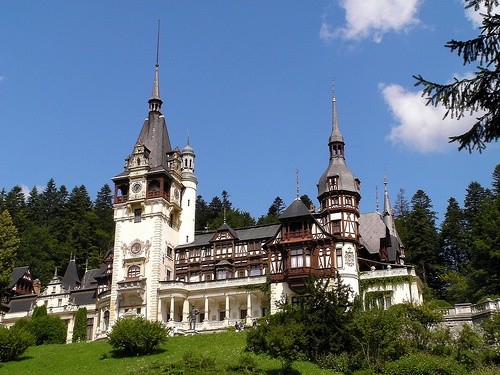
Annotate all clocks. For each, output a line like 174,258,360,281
132,181,143,193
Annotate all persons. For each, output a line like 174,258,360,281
253,319,256,326
235,321,239,330
239,322,244,327
188,306,199,330
